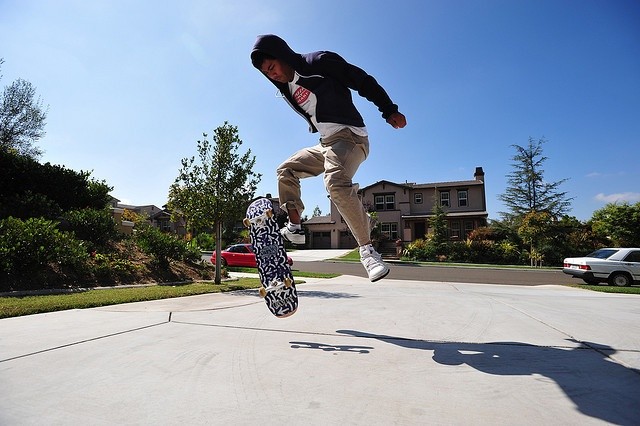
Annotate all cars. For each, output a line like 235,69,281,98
563,248,640,287
210,244,293,269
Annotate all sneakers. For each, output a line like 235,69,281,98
280,226,306,247
360,250,389,282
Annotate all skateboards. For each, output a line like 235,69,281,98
243,198,298,318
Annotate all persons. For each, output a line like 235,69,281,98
249,32,408,283
